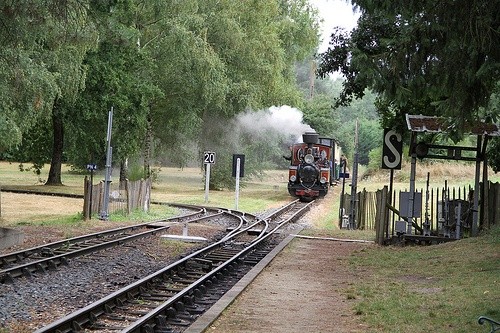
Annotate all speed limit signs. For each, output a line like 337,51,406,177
203,151,216,165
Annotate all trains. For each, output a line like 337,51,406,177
283,131,348,200
